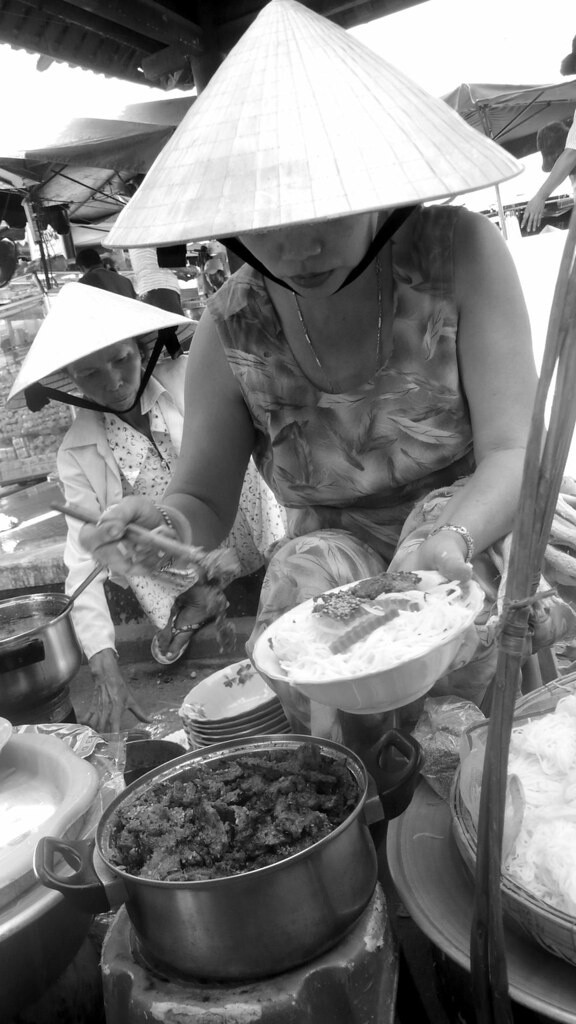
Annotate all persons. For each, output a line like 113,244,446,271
519,35,576,233
56,282,293,735
71,247,138,300
78,0,552,749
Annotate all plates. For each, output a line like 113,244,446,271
0,717,12,753
178,659,294,750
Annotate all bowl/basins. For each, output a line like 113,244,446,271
251,571,486,715
0,734,99,908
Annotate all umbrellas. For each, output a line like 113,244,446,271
425,75,576,242
1,94,197,292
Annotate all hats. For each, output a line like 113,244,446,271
560,35,576,76
6,281,199,405
102,0,525,255
537,120,570,173
204,258,225,275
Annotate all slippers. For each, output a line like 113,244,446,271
150,605,201,666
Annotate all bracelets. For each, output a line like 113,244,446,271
155,506,174,528
425,523,474,562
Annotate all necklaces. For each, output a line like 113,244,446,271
292,254,382,392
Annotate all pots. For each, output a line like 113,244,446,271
34,729,426,979
0,593,82,709
